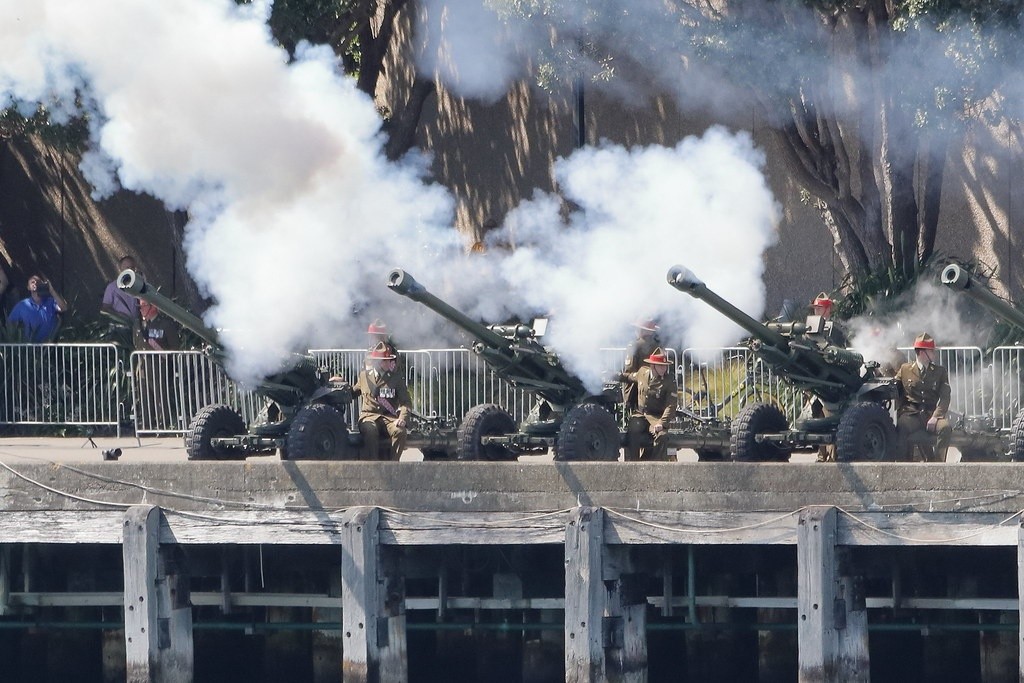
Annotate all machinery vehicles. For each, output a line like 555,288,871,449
666,263,905,468
116,269,354,460
386,264,627,465
941,261,1024,334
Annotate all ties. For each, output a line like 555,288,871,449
649,376,661,384
920,365,926,379
376,373,381,383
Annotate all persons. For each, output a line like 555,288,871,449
101,256,153,345
133,298,179,438
808,292,847,462
0,264,67,344
467,218,513,258
867,327,952,462
597,347,678,462
328,318,412,461
618,317,662,429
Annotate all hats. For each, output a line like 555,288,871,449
808,291,837,308
634,318,660,331
367,341,396,360
365,319,391,335
914,333,941,350
643,347,674,366
